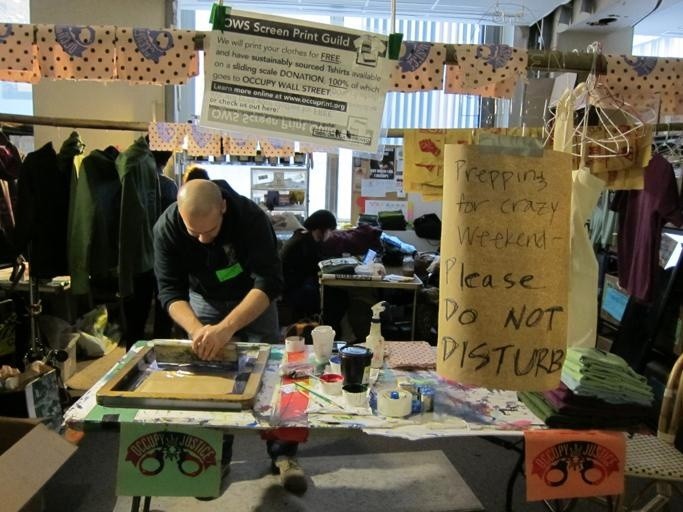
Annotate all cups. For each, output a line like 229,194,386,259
402,255,415,277
284,334,306,362
311,325,336,363
337,344,375,393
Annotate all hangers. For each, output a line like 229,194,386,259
541,42,657,160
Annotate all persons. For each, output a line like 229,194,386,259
279,208,349,344
149,177,308,502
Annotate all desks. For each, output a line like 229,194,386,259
60,340,578,512
317,270,424,343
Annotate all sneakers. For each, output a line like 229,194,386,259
195,464,231,501
271,454,307,495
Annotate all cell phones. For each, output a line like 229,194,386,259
332,340,346,353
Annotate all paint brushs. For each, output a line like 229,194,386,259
291,380,345,410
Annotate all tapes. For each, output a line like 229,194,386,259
376,387,414,417
